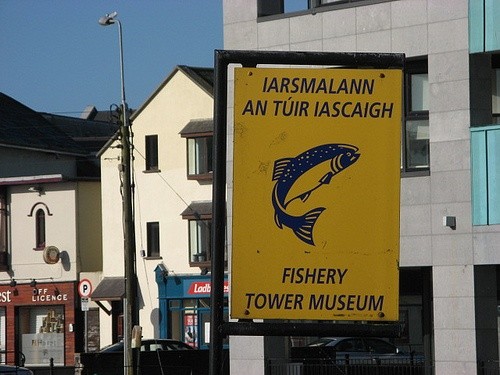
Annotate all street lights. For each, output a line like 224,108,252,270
98,11,139,375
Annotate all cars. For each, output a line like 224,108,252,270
99,338,195,353
308,337,425,367
